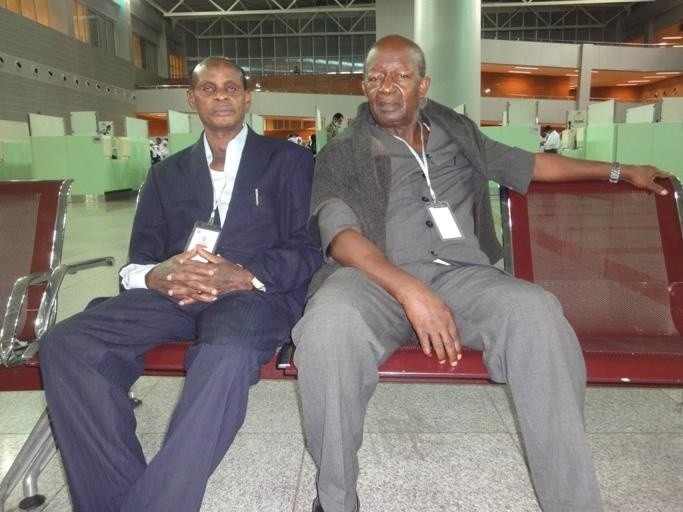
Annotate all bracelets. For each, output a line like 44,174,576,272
605,160,622,184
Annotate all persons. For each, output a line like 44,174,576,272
148,136,169,165
538,123,560,154
326,110,345,141
287,30,675,512
303,133,316,155
37,53,324,511
98,125,111,135
294,133,303,147
286,133,294,144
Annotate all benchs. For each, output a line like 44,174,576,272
1,173,682,508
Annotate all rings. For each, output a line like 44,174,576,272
196,290,204,296
